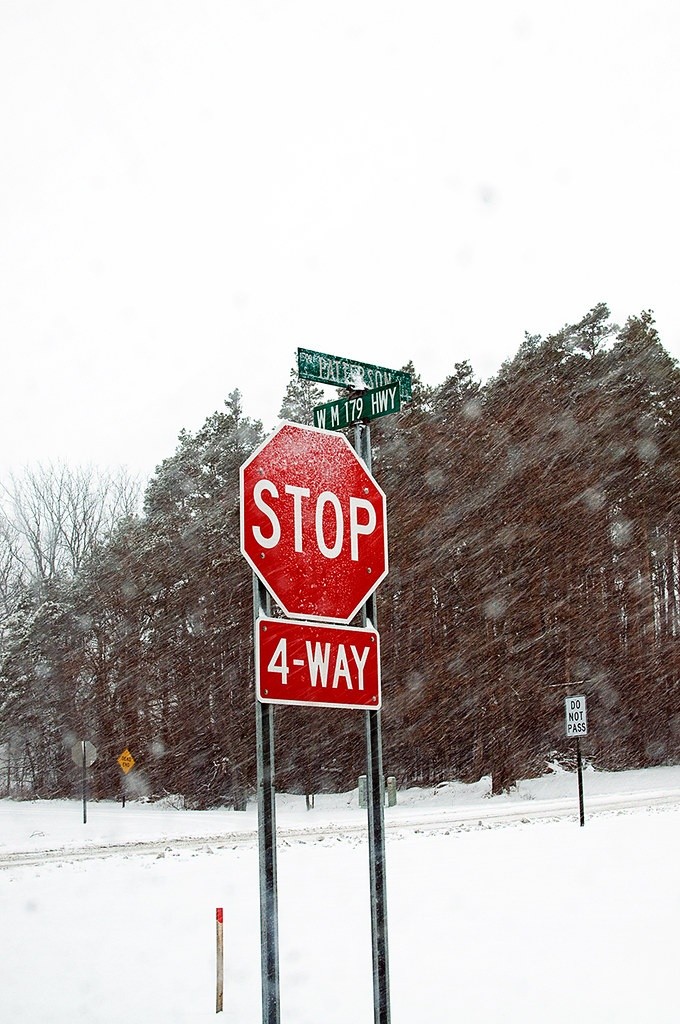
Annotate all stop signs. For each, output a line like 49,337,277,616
237,421,388,712
